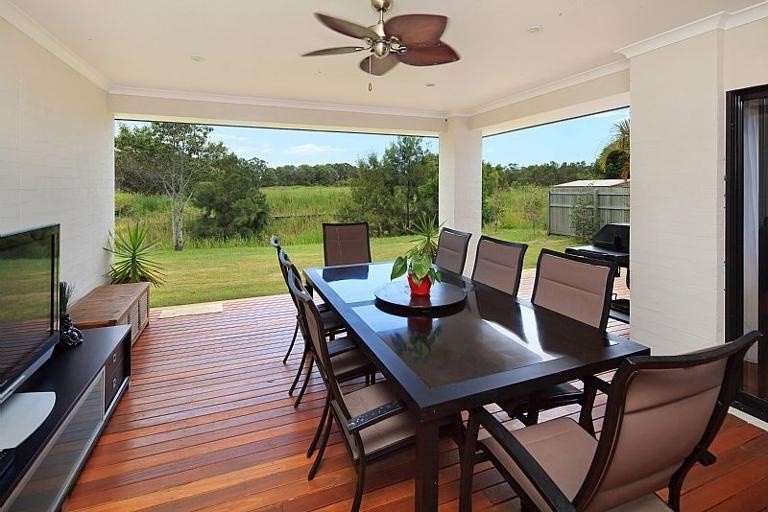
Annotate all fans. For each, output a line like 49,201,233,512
299,0,458,94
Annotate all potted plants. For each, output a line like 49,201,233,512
390,310,443,365
102,218,171,328
390,214,447,297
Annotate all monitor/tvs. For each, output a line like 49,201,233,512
0,222,60,407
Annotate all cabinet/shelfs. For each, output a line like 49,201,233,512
68,281,151,346
0,324,132,512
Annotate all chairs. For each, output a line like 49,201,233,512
433,227,473,276
321,220,372,266
277,248,383,458
286,271,468,512
460,326,765,512
268,234,354,394
469,235,528,295
492,248,619,427
